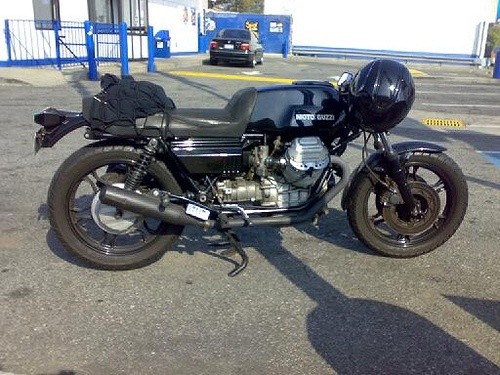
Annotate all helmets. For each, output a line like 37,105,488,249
349,59,415,133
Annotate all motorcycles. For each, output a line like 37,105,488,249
34,58,469,277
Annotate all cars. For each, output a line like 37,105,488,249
209,27,266,69
